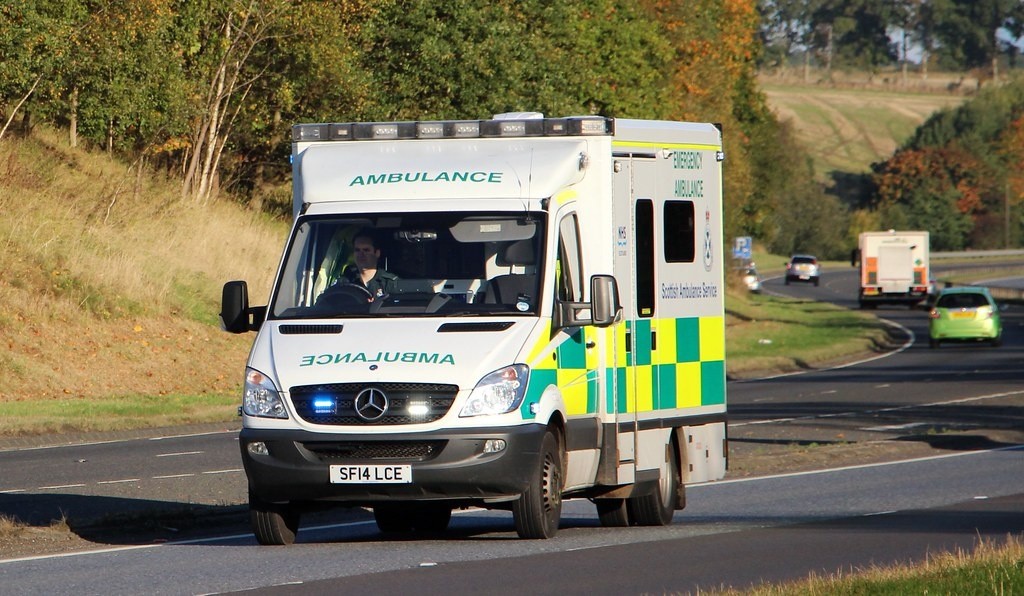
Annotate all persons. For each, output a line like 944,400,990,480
348,227,399,303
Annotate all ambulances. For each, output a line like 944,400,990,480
219,112,729,547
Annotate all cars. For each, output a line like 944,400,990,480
742,266,761,293
783,254,821,287
929,287,1001,349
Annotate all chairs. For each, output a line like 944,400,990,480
483,237,539,306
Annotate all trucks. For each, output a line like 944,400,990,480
857,229,930,309
